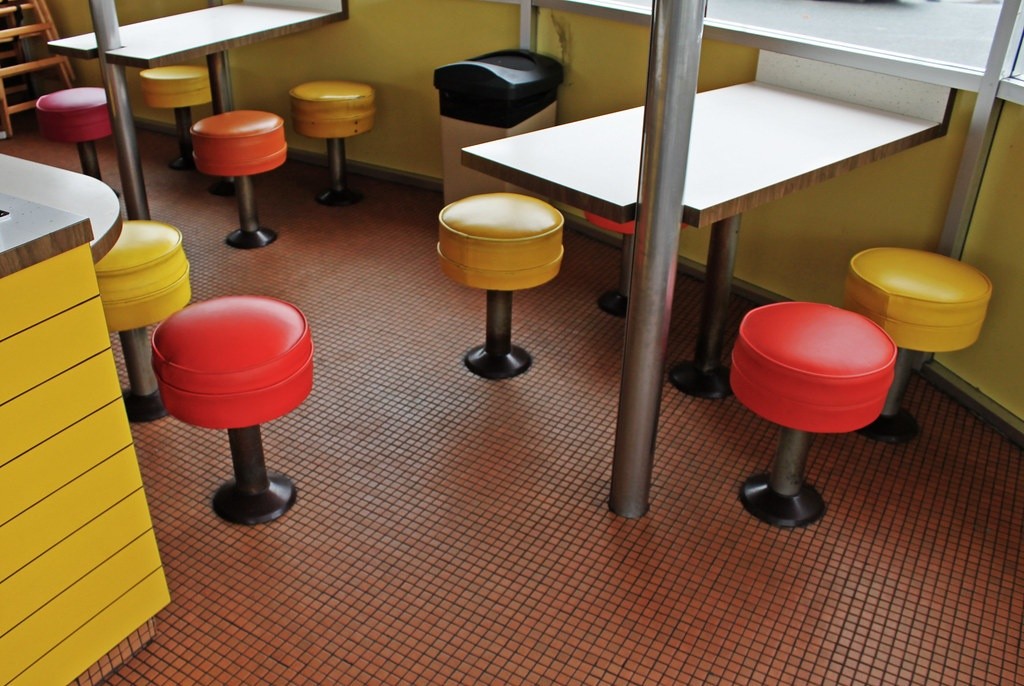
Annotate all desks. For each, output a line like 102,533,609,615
42,1,348,202
457,51,962,405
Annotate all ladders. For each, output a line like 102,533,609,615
0,0,77,137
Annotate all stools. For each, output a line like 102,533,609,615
95,217,195,421
435,190,565,378
149,295,315,524
846,245,993,440
728,299,900,531
190,108,287,248
584,208,691,321
287,76,380,206
32,86,124,199
139,61,214,171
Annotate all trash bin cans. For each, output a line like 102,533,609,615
429,48,567,207
